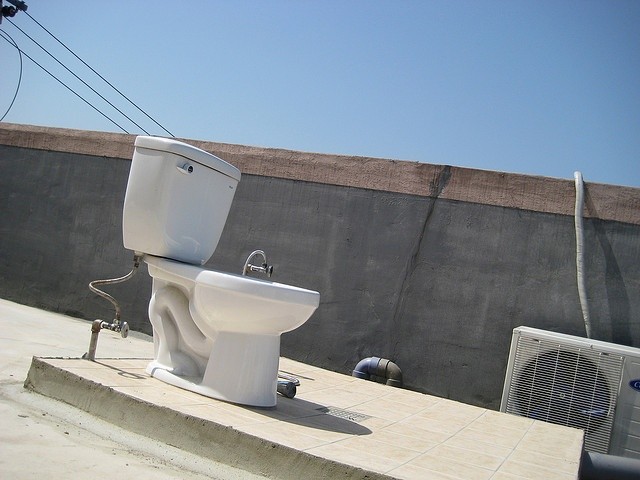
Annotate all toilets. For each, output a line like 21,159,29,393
121,136,321,408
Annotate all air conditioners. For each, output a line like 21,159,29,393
500,325,640,461
